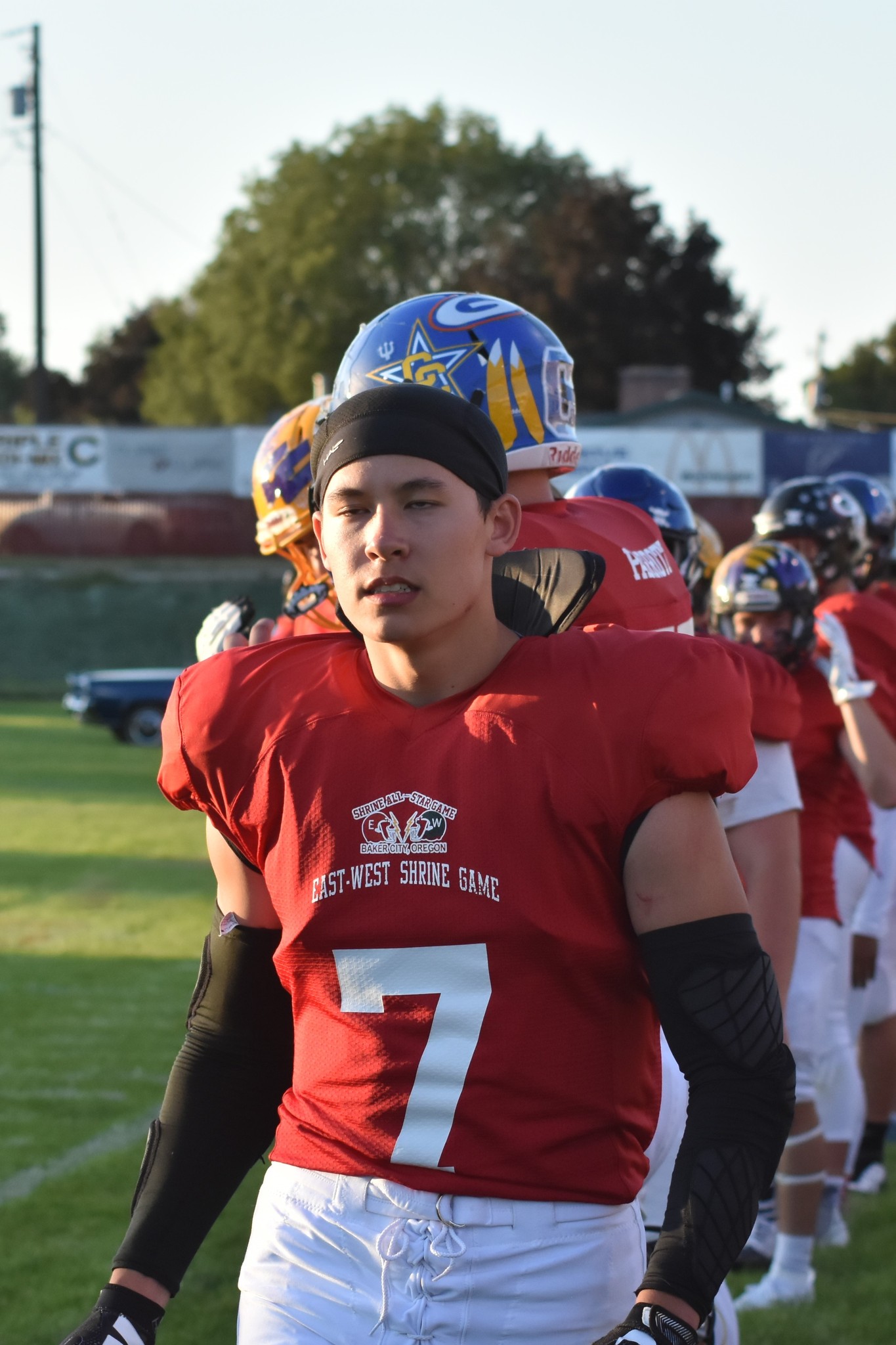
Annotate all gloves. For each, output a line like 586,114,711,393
196,592,256,661
594,1302,714,1344
60,1283,164,1344
814,613,875,704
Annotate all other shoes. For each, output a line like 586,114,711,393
726,1158,885,1310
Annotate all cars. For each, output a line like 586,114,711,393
58,663,198,750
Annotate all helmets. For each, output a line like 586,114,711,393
561,464,702,589
689,515,723,579
313,291,582,476
756,476,873,567
829,473,894,526
251,394,329,555
711,539,818,614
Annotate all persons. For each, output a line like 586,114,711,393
73,289,896,1345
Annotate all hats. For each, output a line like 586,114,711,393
309,380,507,510
